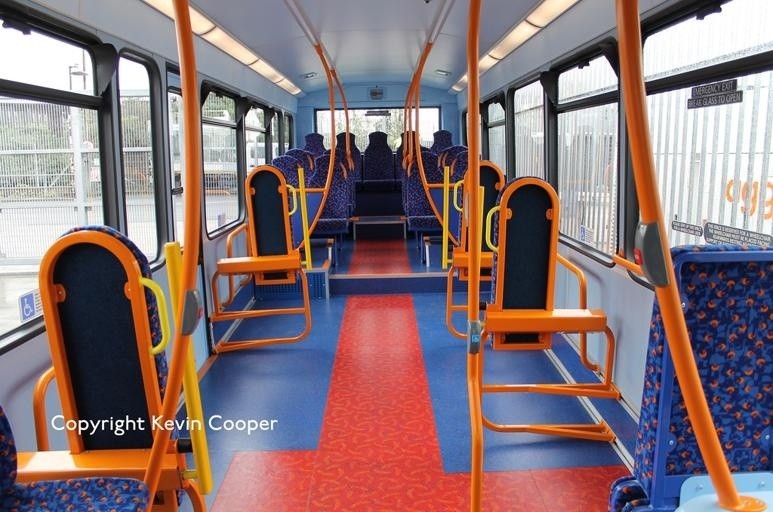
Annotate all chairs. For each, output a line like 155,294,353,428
0,405,149,511
271,130,482,265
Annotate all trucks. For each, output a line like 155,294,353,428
174,141,289,190
